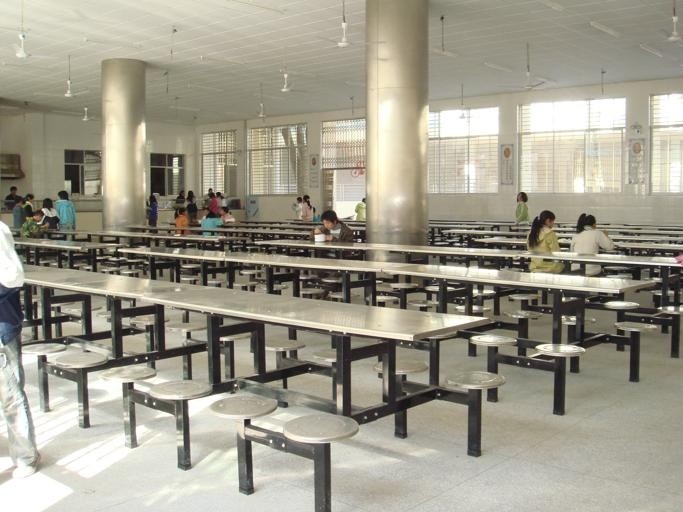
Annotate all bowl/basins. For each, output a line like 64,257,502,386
315,234,326,242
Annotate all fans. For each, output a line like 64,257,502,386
495,42,553,106
34,56,90,104
281,73,291,92
72,108,100,123
10,31,33,59
654,7,683,45
345,96,359,119
317,0,388,53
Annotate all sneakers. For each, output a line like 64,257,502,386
13,453,41,478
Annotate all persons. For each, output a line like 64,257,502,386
527,210,566,273
5,186,76,238
146,188,236,236
569,214,614,277
292,195,366,259
515,192,529,238
0,220,41,479
